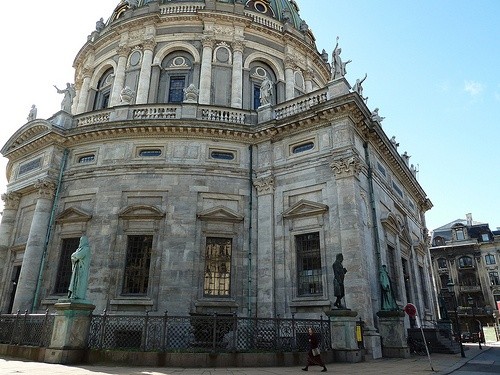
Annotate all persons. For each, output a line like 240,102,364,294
301,328,328,372
333,254,346,309
67,236,92,304
379,264,400,312
